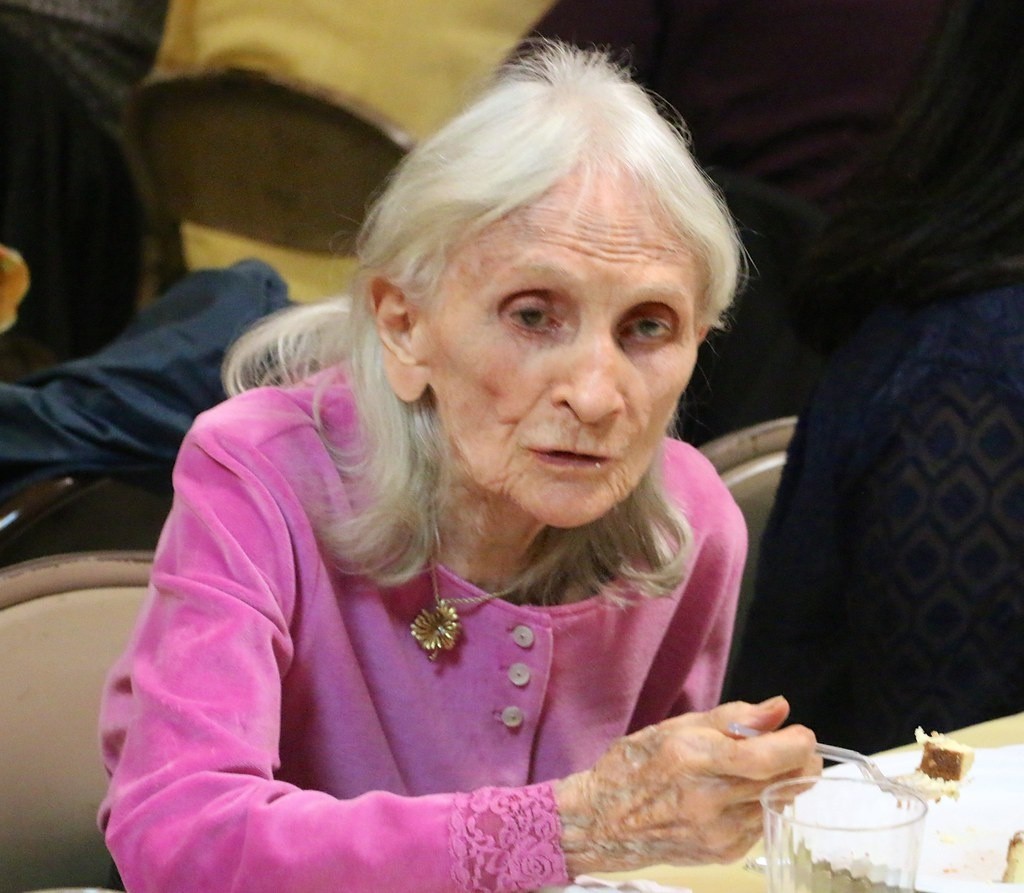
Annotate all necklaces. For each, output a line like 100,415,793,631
410,542,526,661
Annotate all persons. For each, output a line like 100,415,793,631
97,30,824,891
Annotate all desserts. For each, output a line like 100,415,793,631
1000,830,1024,884
897,726,979,798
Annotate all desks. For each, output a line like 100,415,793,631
565,709,1023,890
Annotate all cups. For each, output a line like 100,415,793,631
757,777,926,891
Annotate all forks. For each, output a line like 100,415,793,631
726,721,943,797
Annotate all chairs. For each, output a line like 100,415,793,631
0,547,203,893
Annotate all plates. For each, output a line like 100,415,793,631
789,747,1024,890
